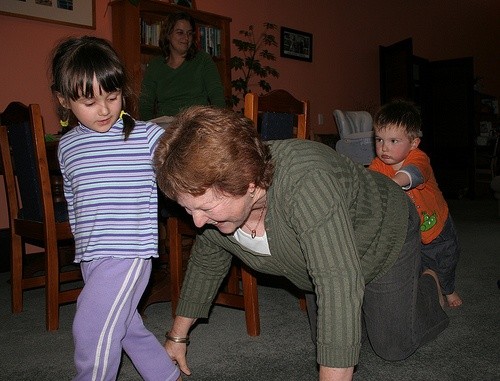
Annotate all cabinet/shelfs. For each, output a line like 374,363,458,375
378,37,475,200
108,0,232,118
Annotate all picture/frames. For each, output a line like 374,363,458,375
280,25,313,63
0,0,97,31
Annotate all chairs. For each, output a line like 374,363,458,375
146,89,341,337
0,99,85,333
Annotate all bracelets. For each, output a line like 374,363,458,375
165,332,190,345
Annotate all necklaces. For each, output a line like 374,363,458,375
244,196,266,239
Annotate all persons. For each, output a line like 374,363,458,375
138,11,228,121
364,99,463,307
46,36,182,381
152,105,449,381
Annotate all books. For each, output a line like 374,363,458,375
194,23,221,57
139,16,166,46
